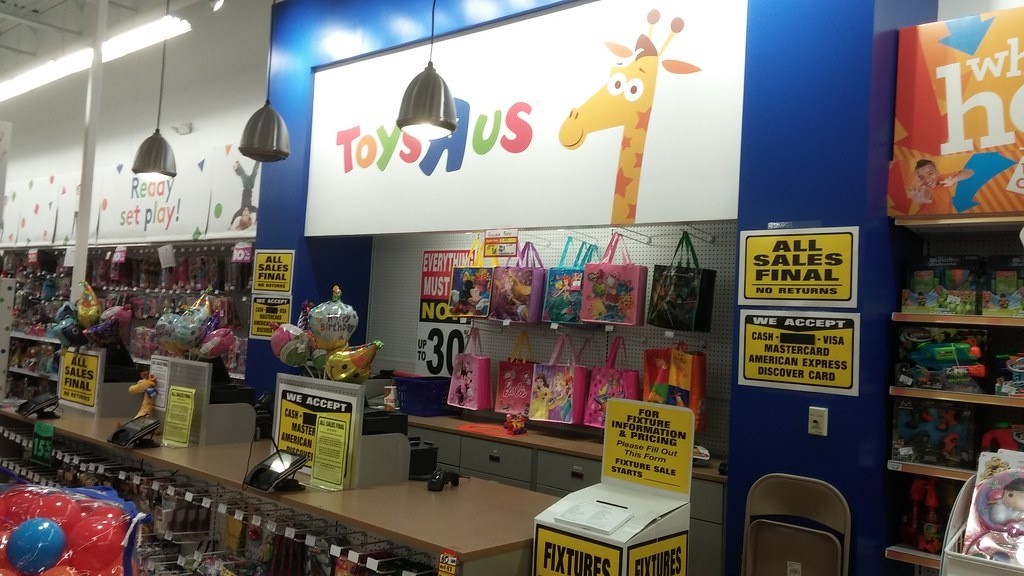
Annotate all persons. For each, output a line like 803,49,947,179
907,159,974,216
227,159,260,230
9,340,58,376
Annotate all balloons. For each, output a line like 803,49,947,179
269,286,384,384
156,294,235,358
53,280,132,348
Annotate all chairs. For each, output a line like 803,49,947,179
743,472,850,576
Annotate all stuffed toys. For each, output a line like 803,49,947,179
114,372,157,430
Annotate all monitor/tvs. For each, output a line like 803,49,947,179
243,450,310,492
18,392,60,417
107,413,161,448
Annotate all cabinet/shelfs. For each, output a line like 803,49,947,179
885,210,1024,576
404,414,727,575
9,330,152,383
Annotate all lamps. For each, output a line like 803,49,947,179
396,0,458,142
132,0,176,184
238,0,288,163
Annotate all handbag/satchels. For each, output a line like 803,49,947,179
447,230,716,433
